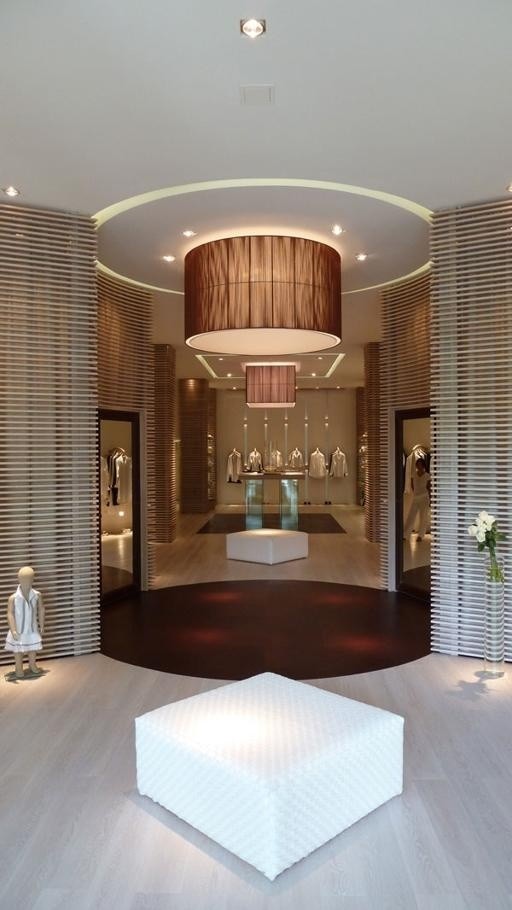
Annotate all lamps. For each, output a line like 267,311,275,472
243,363,301,409
185,234,343,358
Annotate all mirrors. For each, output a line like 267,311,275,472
97,410,141,605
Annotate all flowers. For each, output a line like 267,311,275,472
469,510,507,584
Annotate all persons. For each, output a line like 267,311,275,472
403,458,432,542
5,566,45,677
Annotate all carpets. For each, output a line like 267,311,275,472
100,578,433,679
196,513,348,534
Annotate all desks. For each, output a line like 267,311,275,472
238,471,305,531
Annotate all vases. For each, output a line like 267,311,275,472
483,556,505,676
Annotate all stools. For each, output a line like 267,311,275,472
225,528,307,565
134,668,406,873
